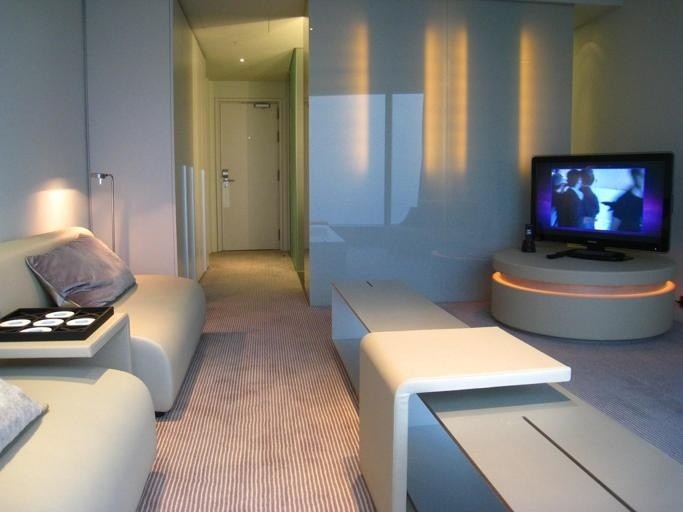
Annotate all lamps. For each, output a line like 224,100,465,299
91,173,115,252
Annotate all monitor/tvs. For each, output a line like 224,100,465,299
530,151,675,262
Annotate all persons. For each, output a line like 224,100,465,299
547,166,600,232
601,169,646,233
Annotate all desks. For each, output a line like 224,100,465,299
330,280,683,512
490,247,676,341
